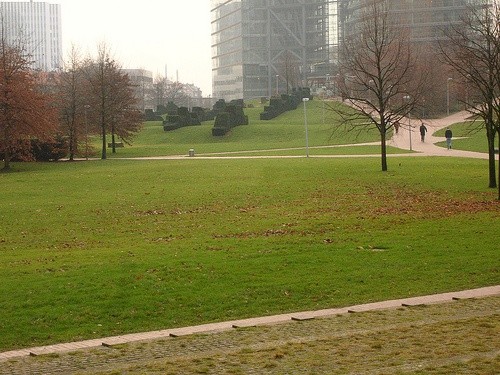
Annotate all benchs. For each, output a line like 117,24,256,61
108,142,124,147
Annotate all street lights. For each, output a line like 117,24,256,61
402,95,413,150
446,77,453,116
368,79,375,97
82,104,91,161
276,74,279,95
465,74,471,109
302,97,310,157
320,86,327,124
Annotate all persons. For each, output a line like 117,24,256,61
394,121,399,133
420,123,427,143
445,127,452,150
